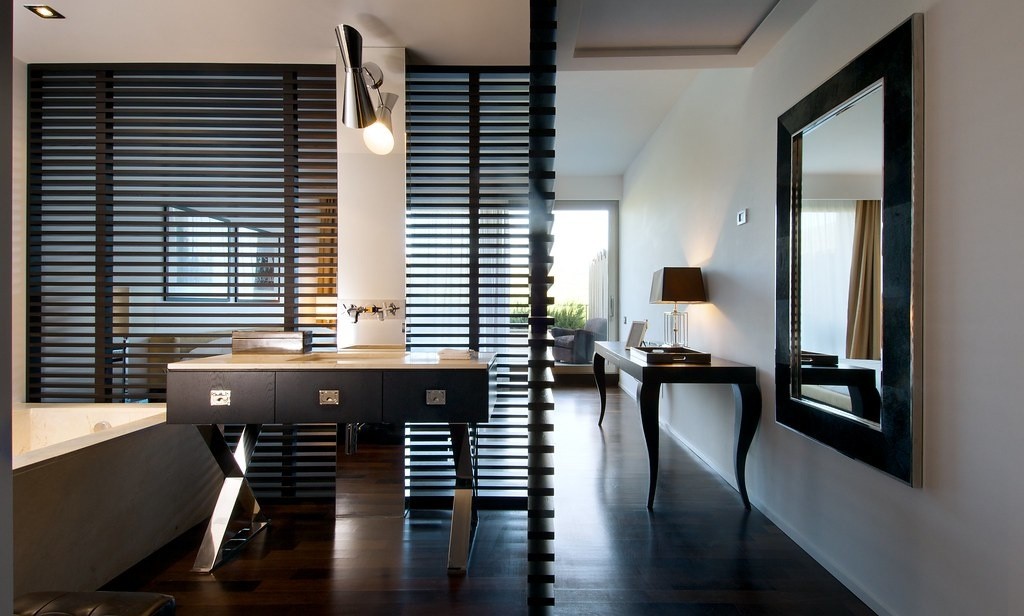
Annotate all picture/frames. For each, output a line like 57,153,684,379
624,320,648,350
165,202,235,300
235,222,281,302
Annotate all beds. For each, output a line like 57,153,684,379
147,324,336,394
797,354,884,416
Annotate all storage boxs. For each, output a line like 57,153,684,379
232,329,314,355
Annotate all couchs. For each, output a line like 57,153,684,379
551,317,608,363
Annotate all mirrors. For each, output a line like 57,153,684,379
336,46,404,520
773,8,931,491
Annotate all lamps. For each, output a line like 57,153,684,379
316,295,338,327
336,24,382,129
363,84,399,155
649,266,707,347
111,285,130,402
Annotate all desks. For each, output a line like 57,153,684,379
802,361,881,424
593,338,762,512
164,349,498,571
